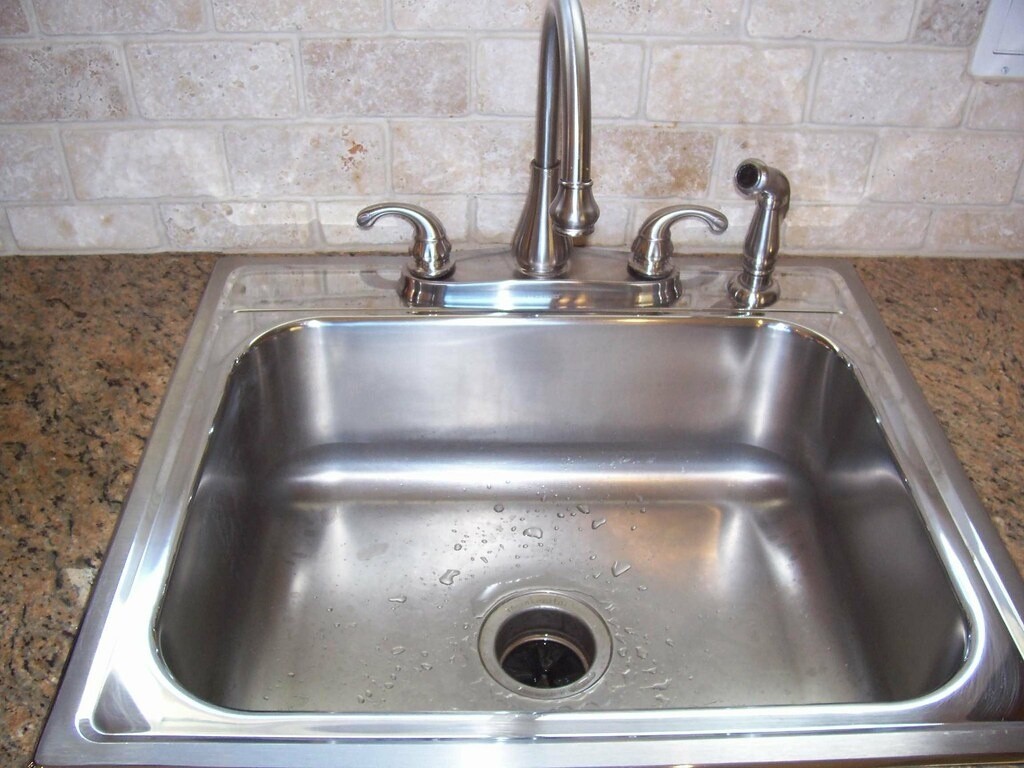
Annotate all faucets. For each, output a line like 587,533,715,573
510,1,600,279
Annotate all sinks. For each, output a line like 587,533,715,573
148,315,985,717
